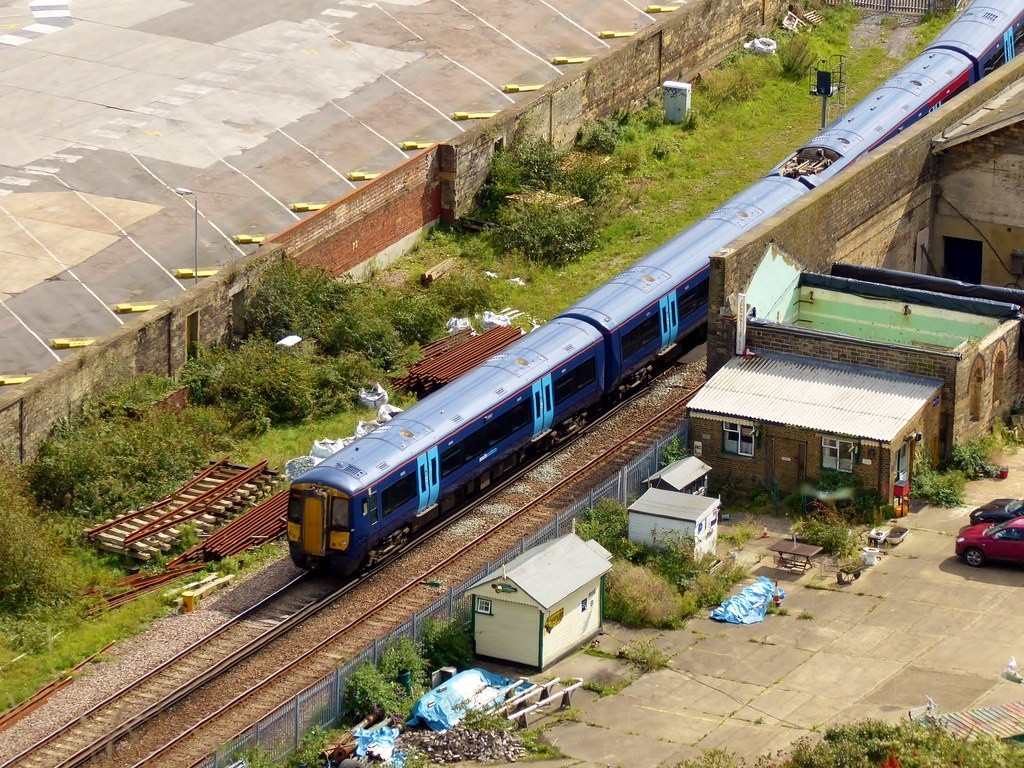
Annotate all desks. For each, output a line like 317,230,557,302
768,540,823,574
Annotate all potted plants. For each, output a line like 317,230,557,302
840,564,865,575
886,526,910,544
998,464,1009,479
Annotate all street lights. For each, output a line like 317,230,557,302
176,186,198,286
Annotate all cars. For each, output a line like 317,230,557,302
955,513,1024,568
970,497,1024,527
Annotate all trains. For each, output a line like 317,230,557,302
287,0,1024,578
